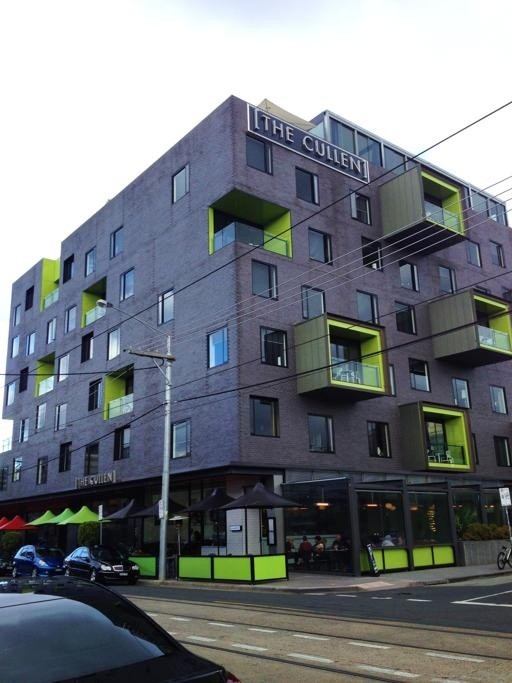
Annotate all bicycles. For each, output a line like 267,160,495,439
497,539,512,569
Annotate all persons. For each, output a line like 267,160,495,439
284,533,403,570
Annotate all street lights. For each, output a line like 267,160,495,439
96,298,176,579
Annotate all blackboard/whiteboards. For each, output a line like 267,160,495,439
366,544,380,575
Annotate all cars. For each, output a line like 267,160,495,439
0,544,140,586
0,575,242,683
